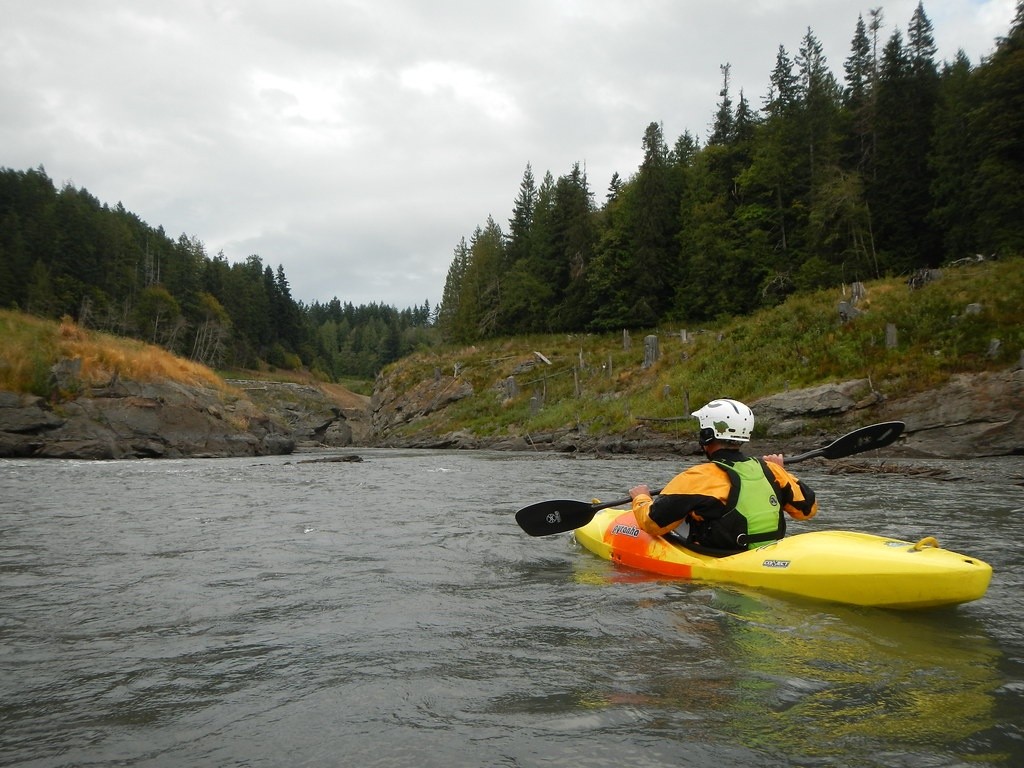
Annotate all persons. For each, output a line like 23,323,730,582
630,398,817,551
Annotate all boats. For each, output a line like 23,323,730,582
572,497,993,609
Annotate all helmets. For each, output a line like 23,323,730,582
691,399,754,441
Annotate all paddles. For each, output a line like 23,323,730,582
513,420,905,540
